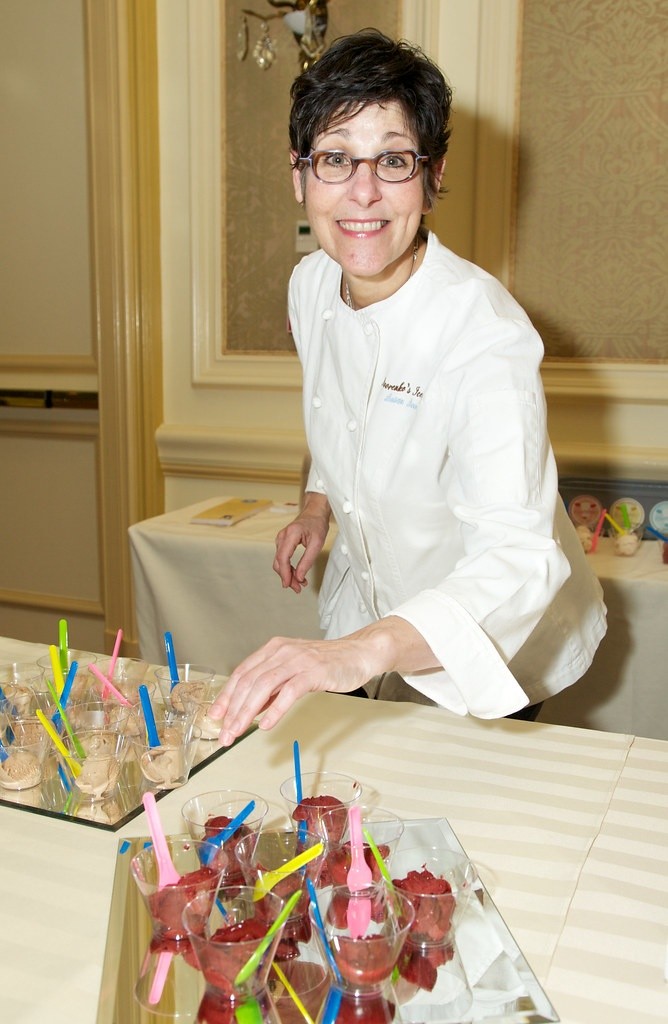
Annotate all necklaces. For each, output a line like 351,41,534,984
344,236,419,308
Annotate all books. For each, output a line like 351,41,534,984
190,497,273,526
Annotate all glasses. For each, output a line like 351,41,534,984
298,149,431,185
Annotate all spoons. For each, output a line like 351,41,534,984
587,504,668,555
0,618,180,792
143,740,402,1024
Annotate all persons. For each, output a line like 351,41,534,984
206,26,607,750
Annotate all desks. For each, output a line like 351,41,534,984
0,634,668,1024
129,497,668,744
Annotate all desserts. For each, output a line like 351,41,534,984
575,522,668,565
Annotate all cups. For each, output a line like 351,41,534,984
0,651,228,802
575,524,605,554
129,769,478,1023
657,529,668,564
607,522,643,556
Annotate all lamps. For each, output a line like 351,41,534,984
236,0,328,75
